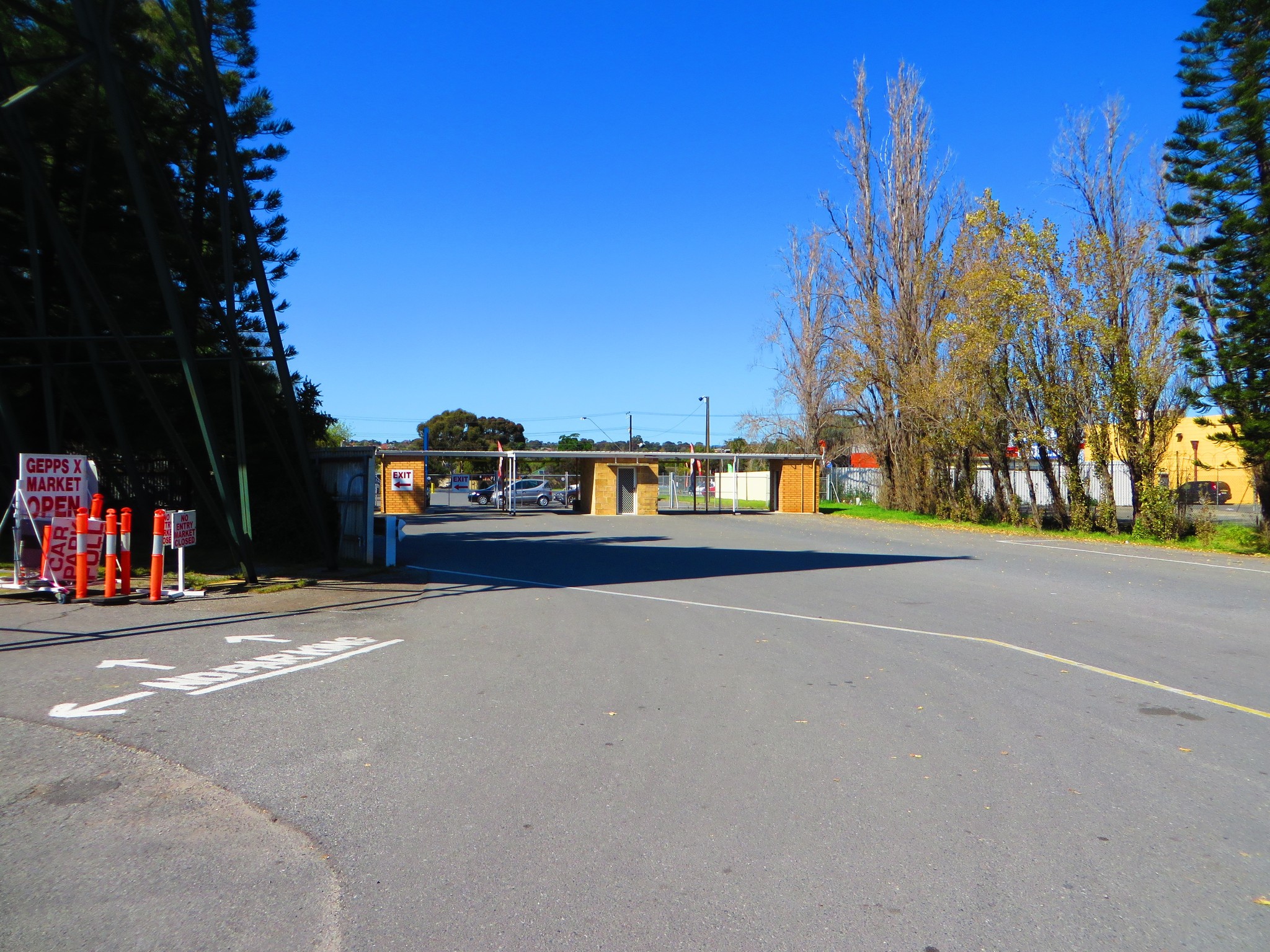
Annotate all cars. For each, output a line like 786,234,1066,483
467,479,514,506
492,478,554,506
555,485,580,504
687,481,716,498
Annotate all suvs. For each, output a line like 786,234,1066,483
1172,480,1230,504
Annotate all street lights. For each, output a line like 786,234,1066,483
583,416,628,453
699,395,711,455
